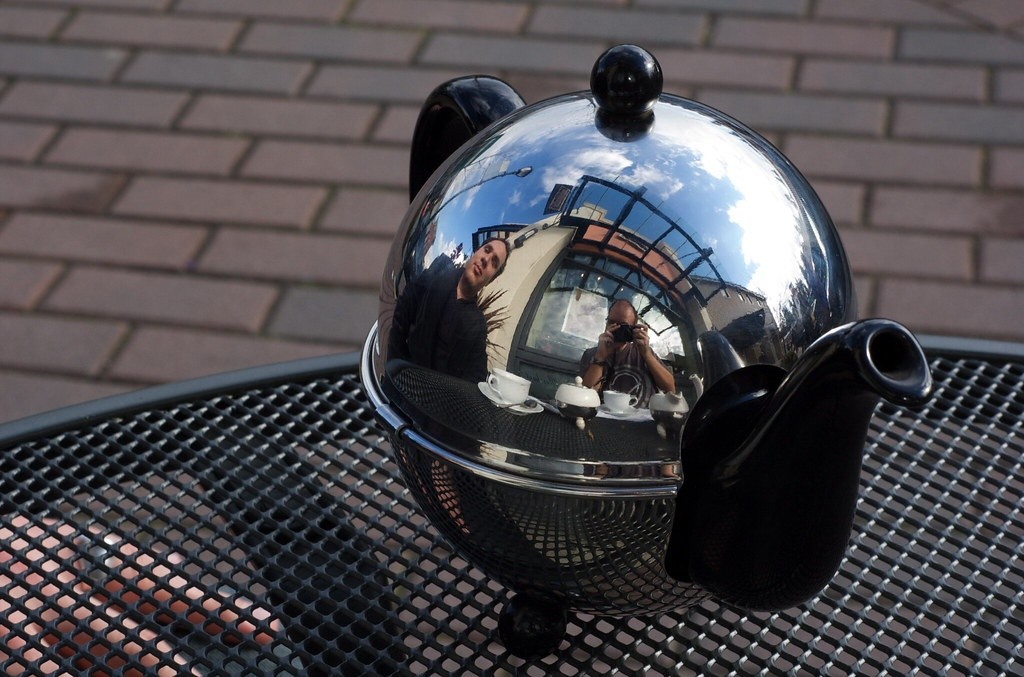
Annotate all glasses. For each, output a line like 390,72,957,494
604,317,629,327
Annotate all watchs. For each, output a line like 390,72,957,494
590,355,606,367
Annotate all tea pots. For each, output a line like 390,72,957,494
355,44,931,623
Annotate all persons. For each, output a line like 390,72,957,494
386,237,509,383
580,298,674,397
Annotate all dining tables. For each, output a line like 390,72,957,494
386,359,680,463
0,332,1024,676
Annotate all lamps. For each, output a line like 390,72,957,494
514,227,538,248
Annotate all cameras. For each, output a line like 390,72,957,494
612,324,641,342
